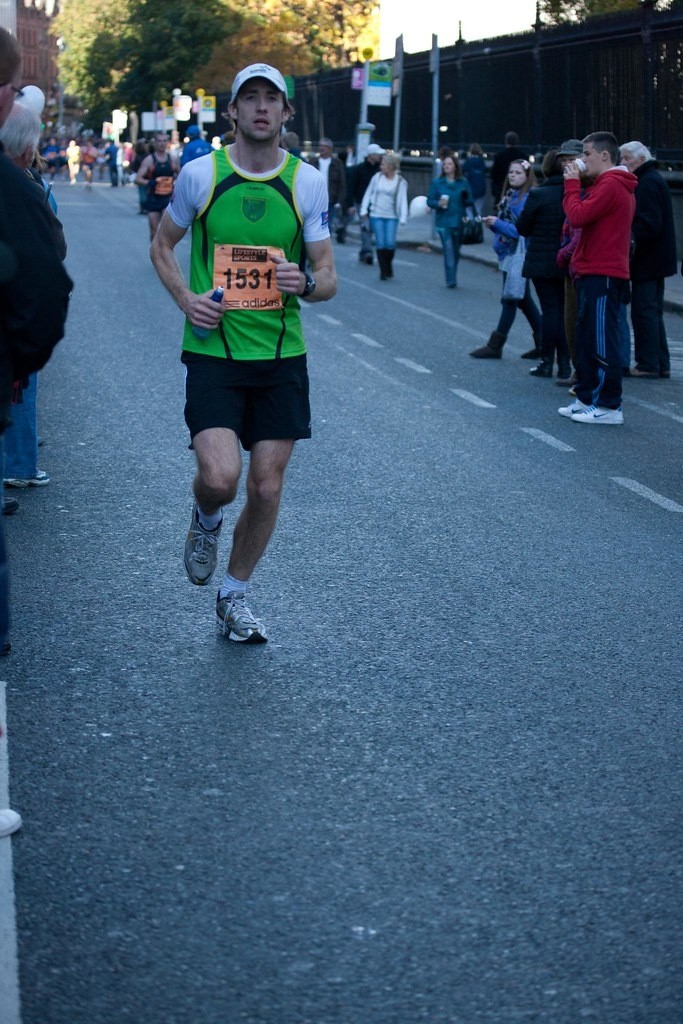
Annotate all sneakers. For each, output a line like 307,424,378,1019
557,399,589,417
571,404,624,424
3,470,49,488
183,500,223,586
216,590,268,643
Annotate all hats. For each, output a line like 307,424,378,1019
367,144,386,155
557,139,583,155
230,62,289,105
185,125,200,137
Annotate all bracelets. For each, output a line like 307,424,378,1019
298,273,310,299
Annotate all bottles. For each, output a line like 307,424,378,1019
192,286,225,338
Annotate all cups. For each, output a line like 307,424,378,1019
441,194,450,208
563,159,586,174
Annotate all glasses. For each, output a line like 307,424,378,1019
1,82,24,100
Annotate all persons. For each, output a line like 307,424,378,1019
463,142,489,245
0,101,42,174
427,154,474,290
150,63,337,644
432,147,453,180
468,159,542,359
309,138,345,241
491,133,522,214
515,149,572,379
555,140,584,386
346,144,388,265
339,142,357,225
280,132,308,165
44,136,133,191
0,27,74,841
620,141,677,379
558,132,638,424
127,126,236,244
359,152,408,281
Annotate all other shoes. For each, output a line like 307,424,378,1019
1,496,19,514
629,366,670,378
554,374,578,386
367,256,373,263
448,284,456,288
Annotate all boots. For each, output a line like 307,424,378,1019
524,334,540,358
557,361,571,378
471,331,507,359
529,357,553,377
377,247,395,280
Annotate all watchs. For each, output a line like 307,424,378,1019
301,274,316,300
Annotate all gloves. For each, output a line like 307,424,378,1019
149,179,157,187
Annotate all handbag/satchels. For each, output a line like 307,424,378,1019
503,236,528,301
459,203,485,245
